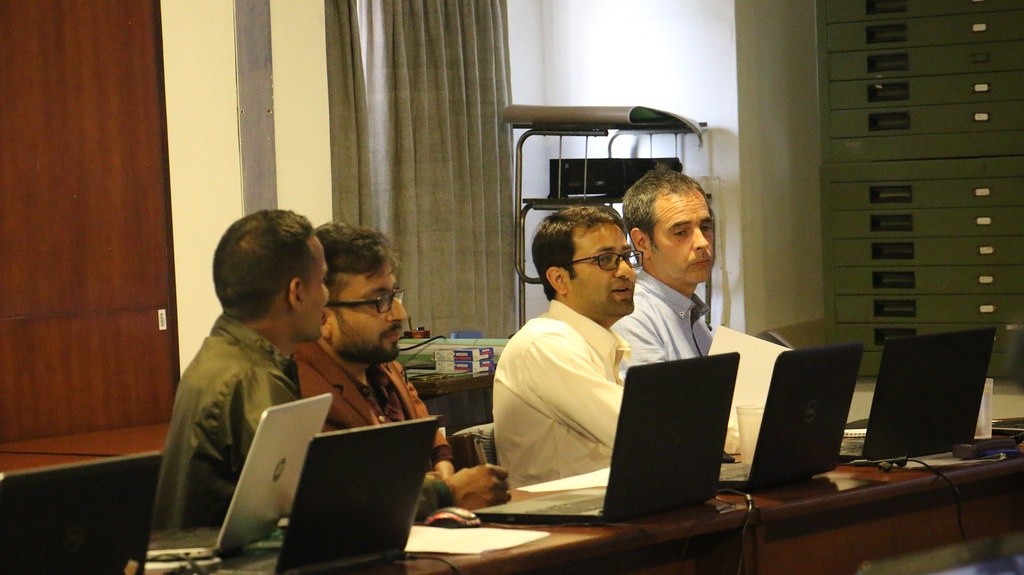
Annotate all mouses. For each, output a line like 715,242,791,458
424,507,481,528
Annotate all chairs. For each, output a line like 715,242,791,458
445,423,493,473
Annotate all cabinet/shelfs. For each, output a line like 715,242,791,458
816,0,1024,379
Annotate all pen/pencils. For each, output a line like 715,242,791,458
474,437,488,466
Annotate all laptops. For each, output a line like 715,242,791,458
473,327,996,523
0,392,443,575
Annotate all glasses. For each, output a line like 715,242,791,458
326,289,406,314
564,251,644,270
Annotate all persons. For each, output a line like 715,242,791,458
150,208,514,526
488,203,647,490
288,219,456,484
604,162,717,383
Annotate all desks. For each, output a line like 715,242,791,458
408,370,494,423
149,417,1023,575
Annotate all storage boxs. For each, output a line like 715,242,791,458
435,347,494,373
